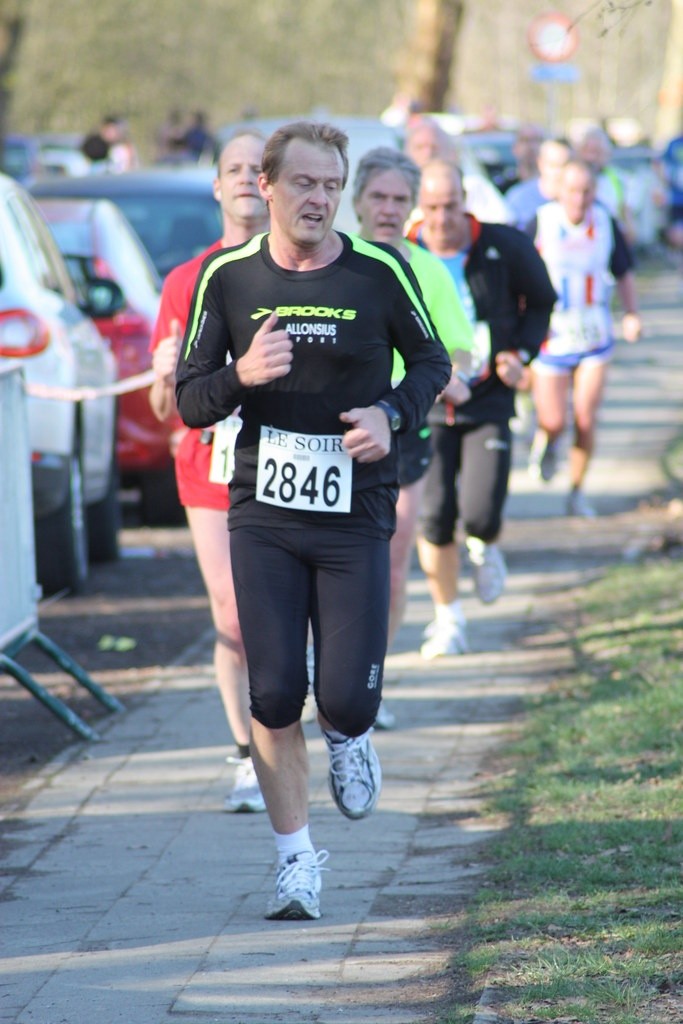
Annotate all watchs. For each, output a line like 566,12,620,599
374,401,401,433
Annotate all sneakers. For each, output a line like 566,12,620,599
304,645,314,684
322,727,381,819
226,756,266,812
265,849,331,920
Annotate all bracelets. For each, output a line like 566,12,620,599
457,371,470,384
509,347,529,364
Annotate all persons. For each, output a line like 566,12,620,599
405,160,559,656
665,134,682,253
149,129,265,811
84,117,118,160
171,110,217,163
381,124,642,523
176,123,454,922
346,147,476,729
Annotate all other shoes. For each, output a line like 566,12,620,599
468,545,505,603
421,620,468,659
375,708,395,729
567,494,595,519
540,444,557,481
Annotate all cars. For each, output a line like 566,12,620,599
213,113,675,254
1,172,127,598
21,166,224,283
25,194,188,530
0,134,89,189
